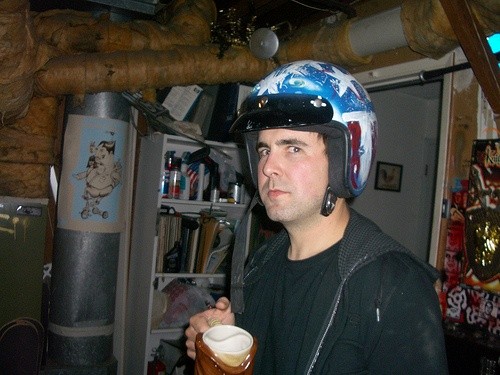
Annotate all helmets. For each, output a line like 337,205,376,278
230,60,379,217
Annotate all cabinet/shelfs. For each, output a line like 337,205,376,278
124,134,252,375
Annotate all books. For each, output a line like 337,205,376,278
158,215,233,274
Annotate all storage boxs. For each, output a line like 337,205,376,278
162,83,253,138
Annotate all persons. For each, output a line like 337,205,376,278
185,60,450,375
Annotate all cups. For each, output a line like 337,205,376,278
228,181,240,202
210,189,220,203
194,317,259,375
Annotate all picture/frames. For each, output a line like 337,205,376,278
373,161,403,193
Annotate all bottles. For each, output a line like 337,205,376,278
162,241,182,273
162,150,190,199
